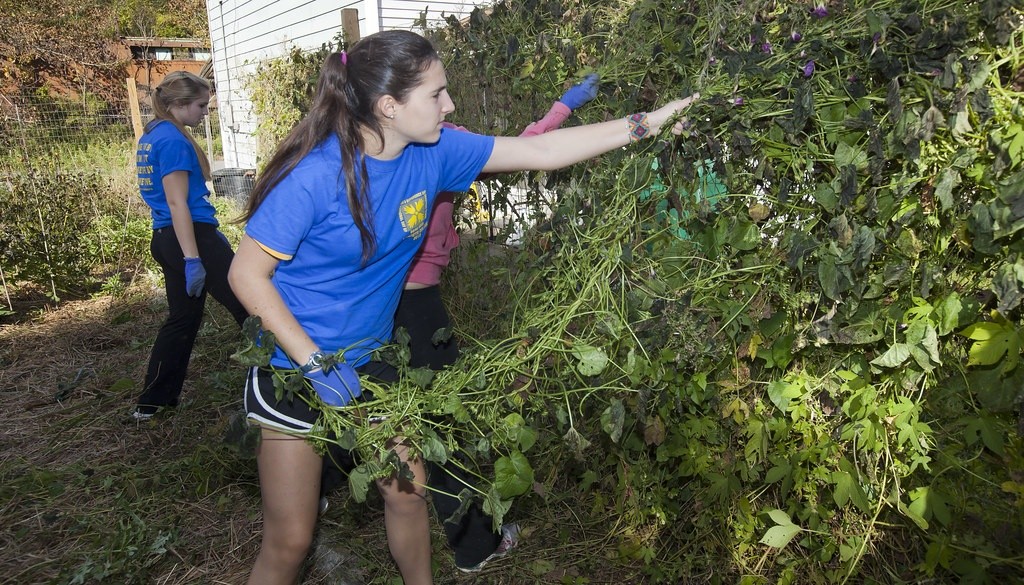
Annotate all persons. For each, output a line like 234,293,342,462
318,74,599,573
132,70,252,422
227,29,700,585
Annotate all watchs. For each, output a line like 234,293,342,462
300,350,325,373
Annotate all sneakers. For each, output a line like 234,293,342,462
319,498,329,515
454,523,521,573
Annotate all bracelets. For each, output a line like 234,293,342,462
624,112,651,145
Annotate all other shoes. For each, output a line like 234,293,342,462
133,404,165,419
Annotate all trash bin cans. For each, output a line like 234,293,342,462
211,166,256,197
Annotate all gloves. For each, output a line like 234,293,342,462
184,257,206,297
304,360,363,411
558,74,600,111
